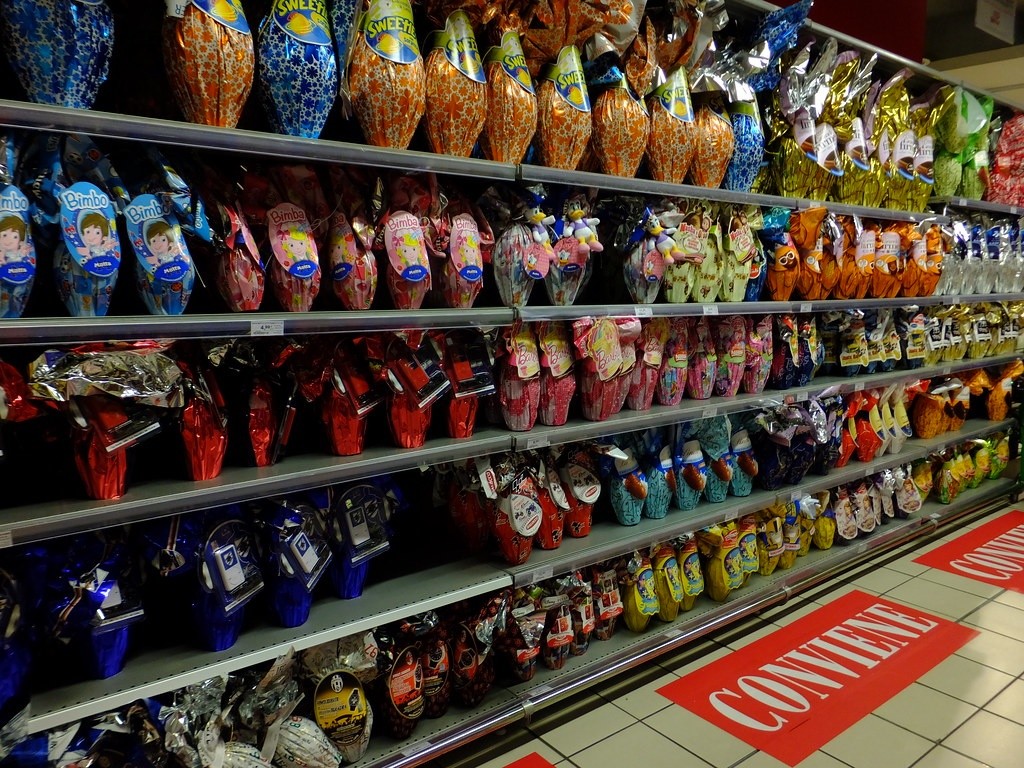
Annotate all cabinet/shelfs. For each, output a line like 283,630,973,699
1,0,1024,768
